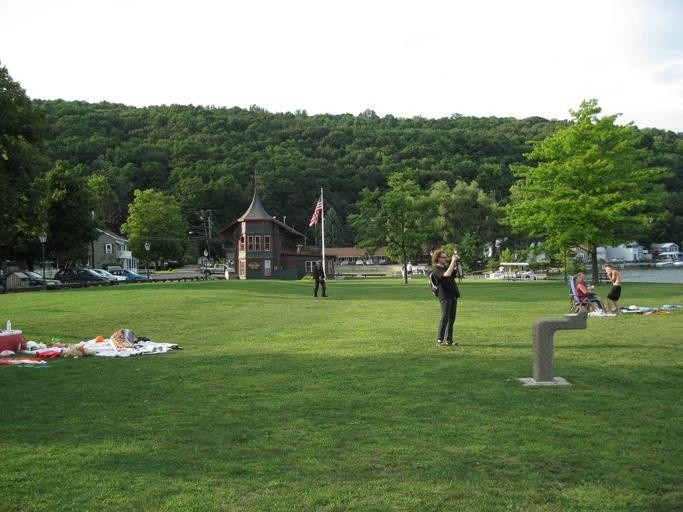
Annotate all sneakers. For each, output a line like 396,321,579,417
436,340,459,346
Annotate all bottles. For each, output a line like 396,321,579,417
7,320,11,333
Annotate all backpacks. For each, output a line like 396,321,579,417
428,271,440,297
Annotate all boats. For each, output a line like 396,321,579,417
655,251,682,267
499,263,534,280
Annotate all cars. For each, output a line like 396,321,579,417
0,268,148,286
379,260,386,265
356,260,364,266
366,260,374,265
341,260,350,265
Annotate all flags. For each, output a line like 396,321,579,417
306,195,322,227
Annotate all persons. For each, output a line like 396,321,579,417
603,264,621,313
574,272,605,312
311,261,328,298
406,261,413,281
400,263,405,280
430,248,463,348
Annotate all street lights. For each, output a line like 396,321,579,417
38,232,48,282
145,241,151,279
204,248,208,280
189,231,210,264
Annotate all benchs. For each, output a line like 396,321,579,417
362,274,386,278
334,273,357,278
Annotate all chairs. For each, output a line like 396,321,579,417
568,275,606,314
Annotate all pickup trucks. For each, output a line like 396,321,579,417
200,264,235,276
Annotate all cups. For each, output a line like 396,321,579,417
126,331,134,343
